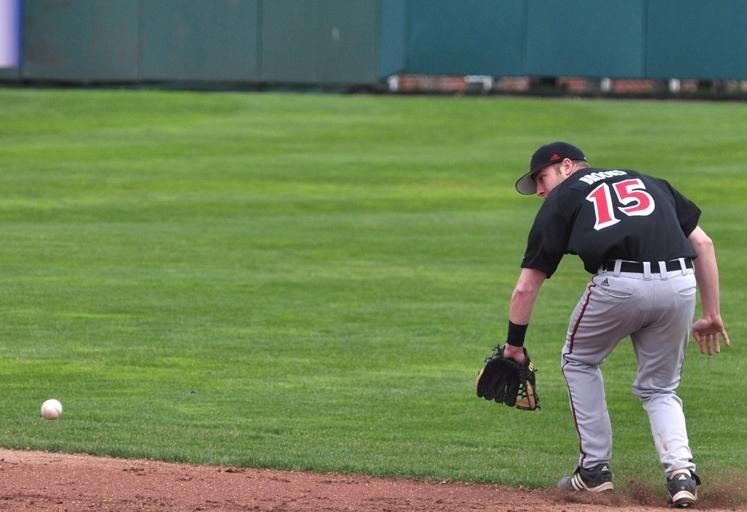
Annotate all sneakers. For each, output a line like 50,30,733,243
556,463,615,495
665,470,702,506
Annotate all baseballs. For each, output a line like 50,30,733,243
40,398,62,419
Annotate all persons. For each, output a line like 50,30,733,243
474,142,731,509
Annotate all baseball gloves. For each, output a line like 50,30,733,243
476,344,540,410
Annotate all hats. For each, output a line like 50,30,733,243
514,143,588,195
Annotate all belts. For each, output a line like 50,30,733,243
600,259,692,275
